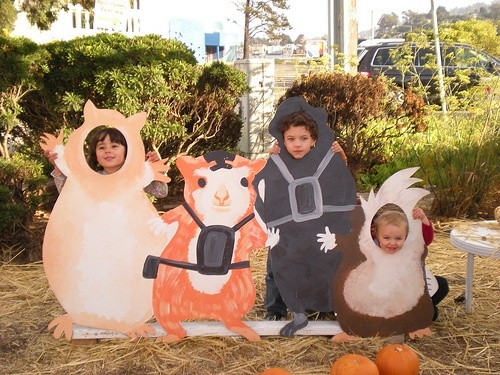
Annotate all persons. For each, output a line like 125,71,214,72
358,197,449,322
44,128,169,198
266,110,348,165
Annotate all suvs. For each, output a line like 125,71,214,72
358,38,500,111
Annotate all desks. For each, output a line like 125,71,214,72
447,225,500,314
